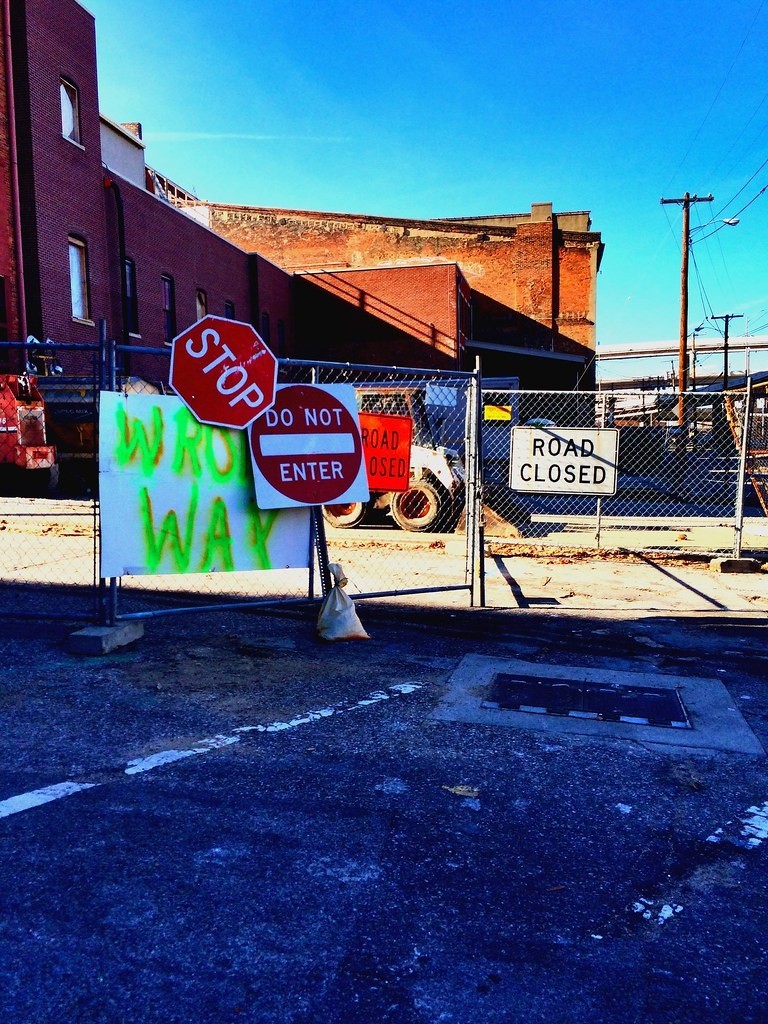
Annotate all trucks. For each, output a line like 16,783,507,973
352,376,519,488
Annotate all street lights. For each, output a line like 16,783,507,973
695,327,728,390
677,219,738,454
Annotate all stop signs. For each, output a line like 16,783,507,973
169,314,278,430
247,383,369,510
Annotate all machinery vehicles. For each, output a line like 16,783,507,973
323,385,466,534
